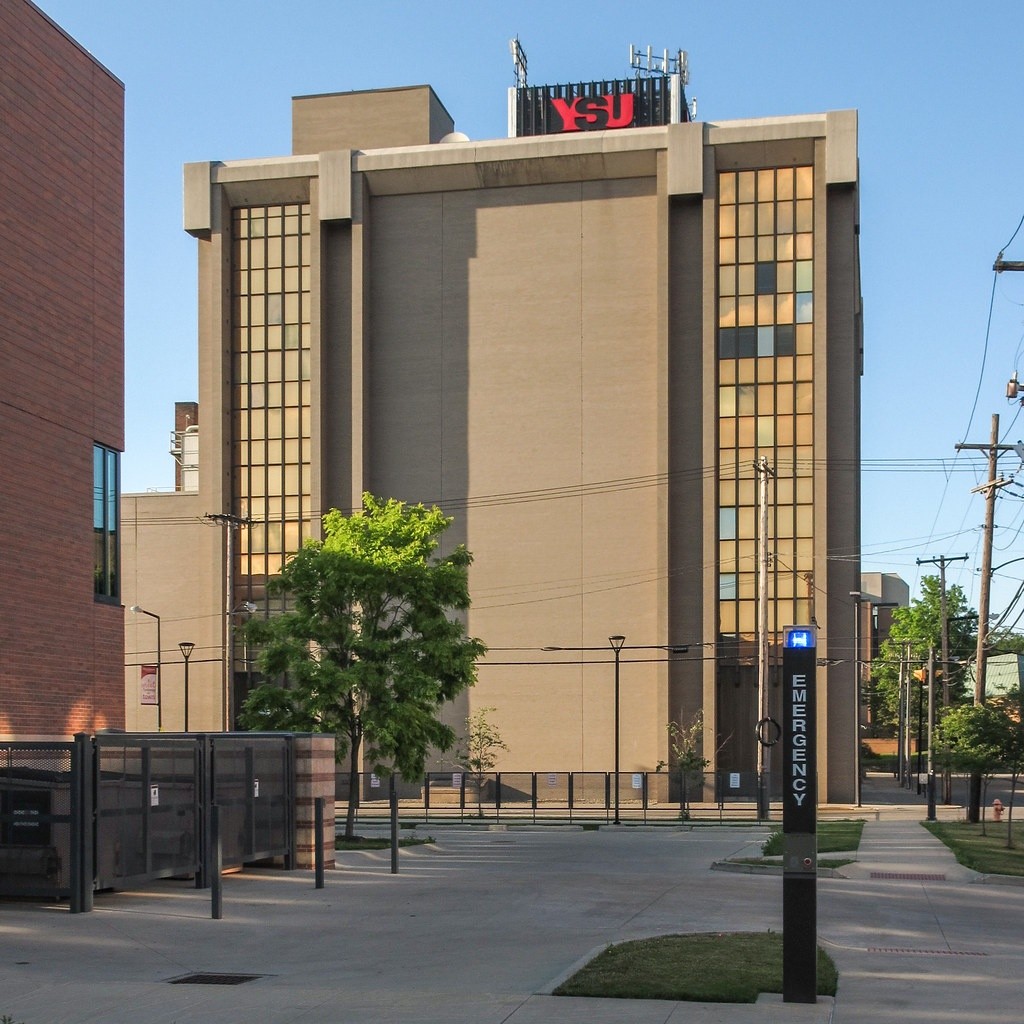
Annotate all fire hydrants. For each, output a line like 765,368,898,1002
991,798,1005,822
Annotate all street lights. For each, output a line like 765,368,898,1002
225,600,258,734
130,605,162,733
178,642,195,731
608,635,628,824
914,667,927,794
849,591,871,808
